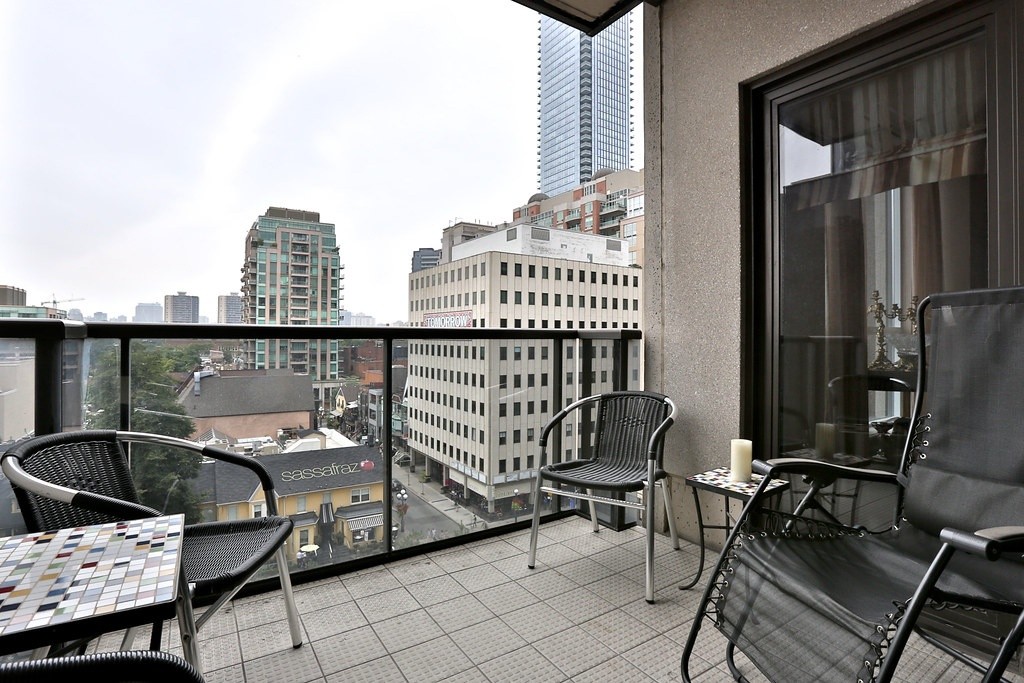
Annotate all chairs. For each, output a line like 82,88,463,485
0,429,302,676
527,391,680,603
828,375,913,530
681,286,1024,683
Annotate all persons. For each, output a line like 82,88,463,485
472,515,477,528
296,550,307,566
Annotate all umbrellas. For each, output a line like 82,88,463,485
301,544,320,552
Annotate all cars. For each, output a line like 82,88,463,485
393,478,407,492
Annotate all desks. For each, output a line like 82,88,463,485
780,448,871,533
0,513,203,676
678,465,790,624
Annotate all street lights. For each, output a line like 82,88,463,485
318,406,325,427
396,488,409,532
514,488,518,522
